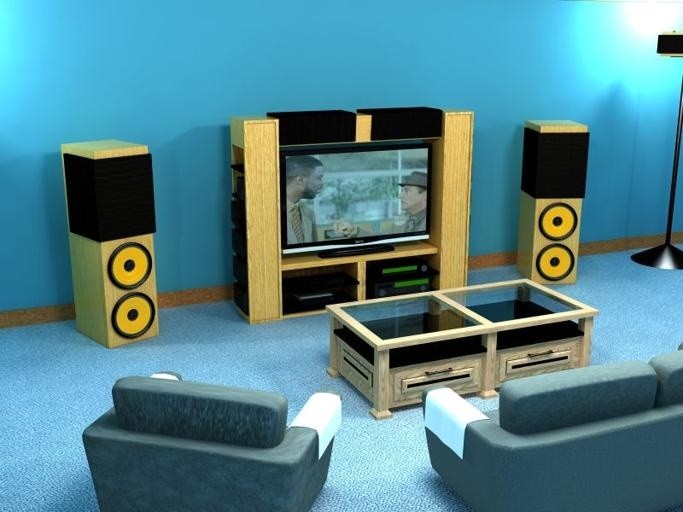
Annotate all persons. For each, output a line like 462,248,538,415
285,157,324,246
332,170,427,237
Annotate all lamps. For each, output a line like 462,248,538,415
632,32,683,271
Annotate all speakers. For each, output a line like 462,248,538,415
516,120,589,283
60,139,159,348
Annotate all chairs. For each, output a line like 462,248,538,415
79,370,342,512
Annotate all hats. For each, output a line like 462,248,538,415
397,170,428,188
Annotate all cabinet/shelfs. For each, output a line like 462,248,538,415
282,244,439,318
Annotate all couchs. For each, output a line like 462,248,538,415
422,340,683,512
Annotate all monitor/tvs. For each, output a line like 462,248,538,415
279,142,432,258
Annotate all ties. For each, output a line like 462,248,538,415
291,205,305,244
